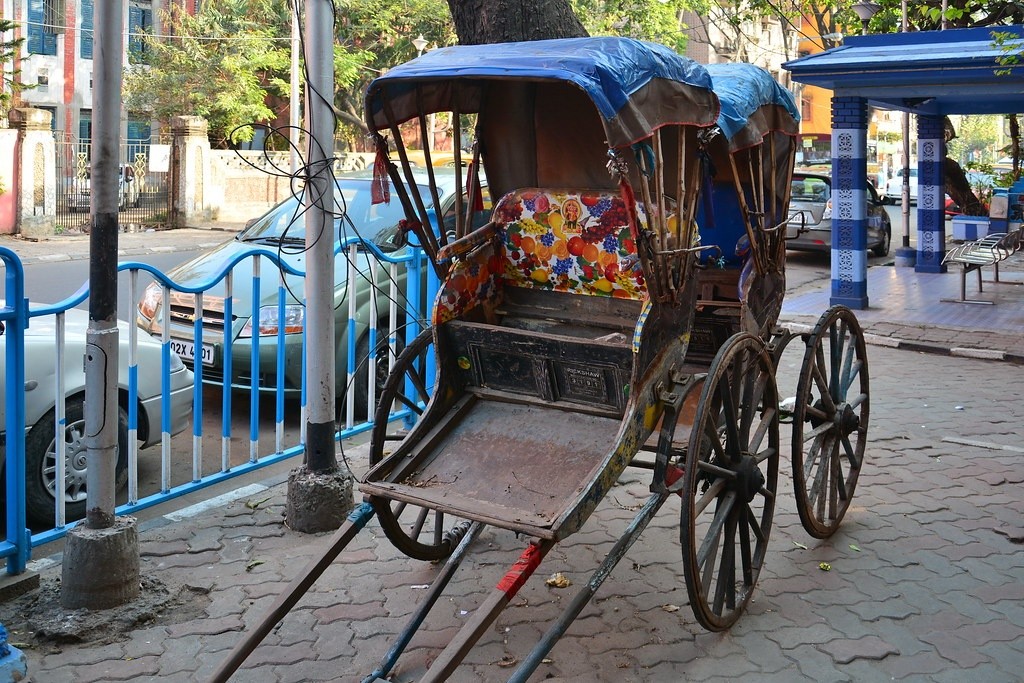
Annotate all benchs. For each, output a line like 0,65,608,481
432,187,699,416
689,184,782,361
941,225,1024,305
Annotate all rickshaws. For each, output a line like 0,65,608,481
200,36,874,682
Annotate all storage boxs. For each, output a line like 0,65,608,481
952,215,991,240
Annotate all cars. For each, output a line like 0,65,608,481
886,168,918,206
352,149,494,213
0,298,197,524
943,164,1024,220
134,161,494,423
786,166,892,258
